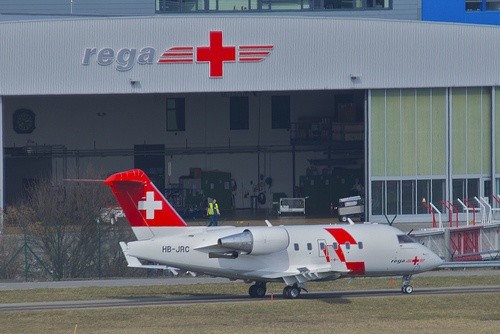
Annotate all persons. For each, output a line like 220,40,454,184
207,198,220,227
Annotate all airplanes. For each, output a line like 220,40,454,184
103,168,445,299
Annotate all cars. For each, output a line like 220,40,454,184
337,194,365,223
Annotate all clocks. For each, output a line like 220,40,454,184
13,109,35,134
